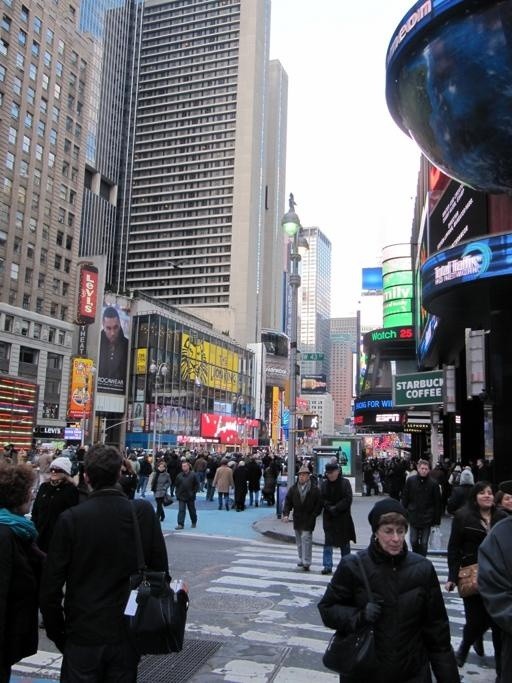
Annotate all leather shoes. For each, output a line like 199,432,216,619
455,639,471,669
472,635,485,657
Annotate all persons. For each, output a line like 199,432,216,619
0,443,512,683
97,308,128,395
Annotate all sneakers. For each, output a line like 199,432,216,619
296,561,310,572
321,567,333,575
175,521,197,530
217,502,259,512
160,515,165,522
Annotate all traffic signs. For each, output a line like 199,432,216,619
301,352,324,361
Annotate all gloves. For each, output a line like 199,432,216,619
361,600,384,623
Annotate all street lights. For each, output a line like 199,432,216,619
77,362,99,448
230,393,245,453
148,361,171,473
279,193,310,524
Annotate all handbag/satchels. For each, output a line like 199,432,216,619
320,622,380,679
457,562,483,597
429,526,443,549
126,569,190,656
163,493,173,506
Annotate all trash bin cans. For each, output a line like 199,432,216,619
276,476,299,519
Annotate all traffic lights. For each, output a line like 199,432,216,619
304,414,319,430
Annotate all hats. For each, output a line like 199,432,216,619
219,456,246,468
50,456,73,477
369,499,410,532
436,458,472,471
325,464,339,474
460,469,475,486
296,464,309,475
